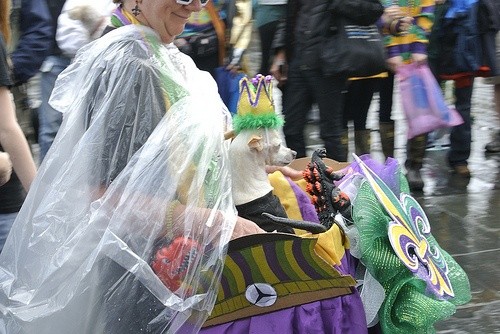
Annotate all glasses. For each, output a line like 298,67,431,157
176,0,210,6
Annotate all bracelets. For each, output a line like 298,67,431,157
164,198,183,241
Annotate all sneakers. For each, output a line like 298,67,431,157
486,139,500,152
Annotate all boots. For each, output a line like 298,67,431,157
355,128,371,156
404,135,426,190
379,120,394,160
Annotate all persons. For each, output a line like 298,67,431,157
0,0,277,334
0,0,500,193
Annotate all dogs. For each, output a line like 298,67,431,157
229,126,297,233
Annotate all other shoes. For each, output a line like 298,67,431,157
450,161,470,176
